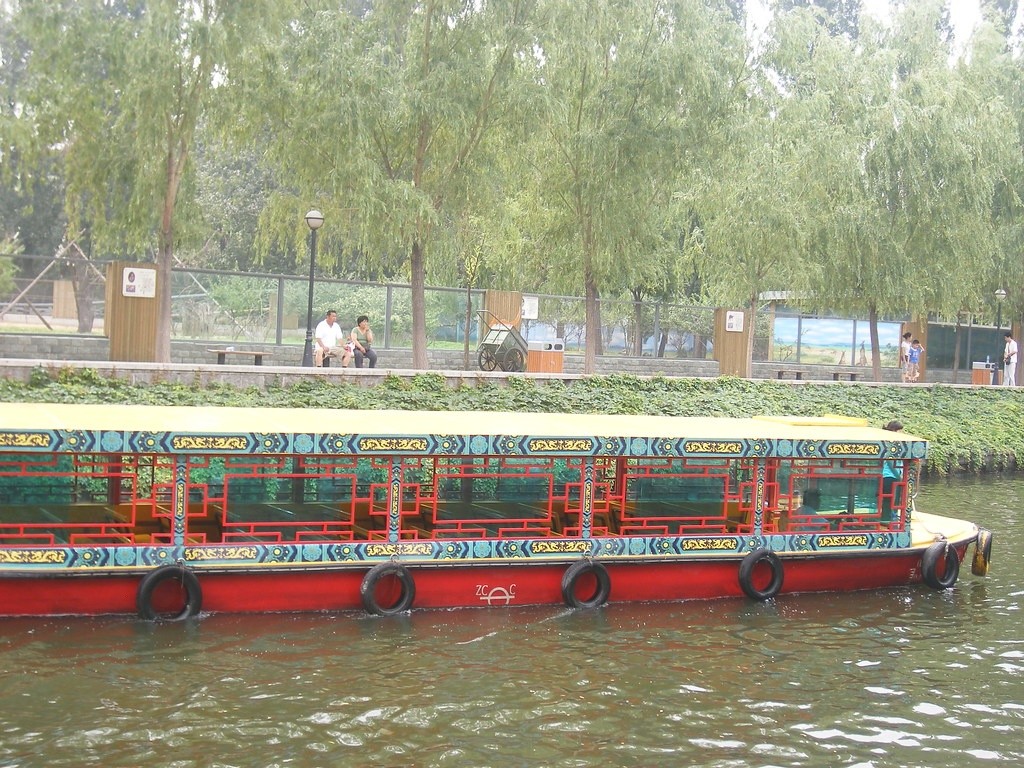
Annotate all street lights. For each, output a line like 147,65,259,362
302,209,326,366
992,288,1007,385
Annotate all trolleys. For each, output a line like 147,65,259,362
473,309,530,372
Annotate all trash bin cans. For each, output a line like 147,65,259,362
971,361,996,385
526,338,565,373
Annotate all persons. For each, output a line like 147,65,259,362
901,332,925,383
1003,332,1018,386
351,316,377,368
794,488,852,531
314,310,351,367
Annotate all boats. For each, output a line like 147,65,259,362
0,400,996,620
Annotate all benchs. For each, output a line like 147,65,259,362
774,369,810,380
208,349,274,366
831,372,863,381
0,500,857,550
305,354,369,368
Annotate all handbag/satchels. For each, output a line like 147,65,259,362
1004,353,1012,365
